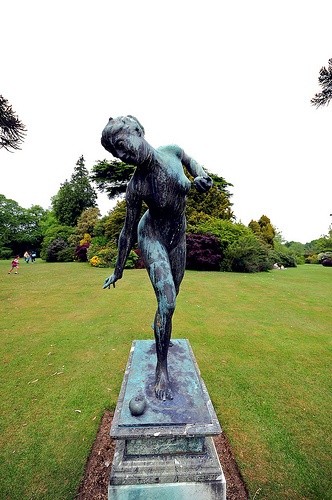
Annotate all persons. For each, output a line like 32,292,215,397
100,116,214,402
8,255,20,274
24,250,37,262
